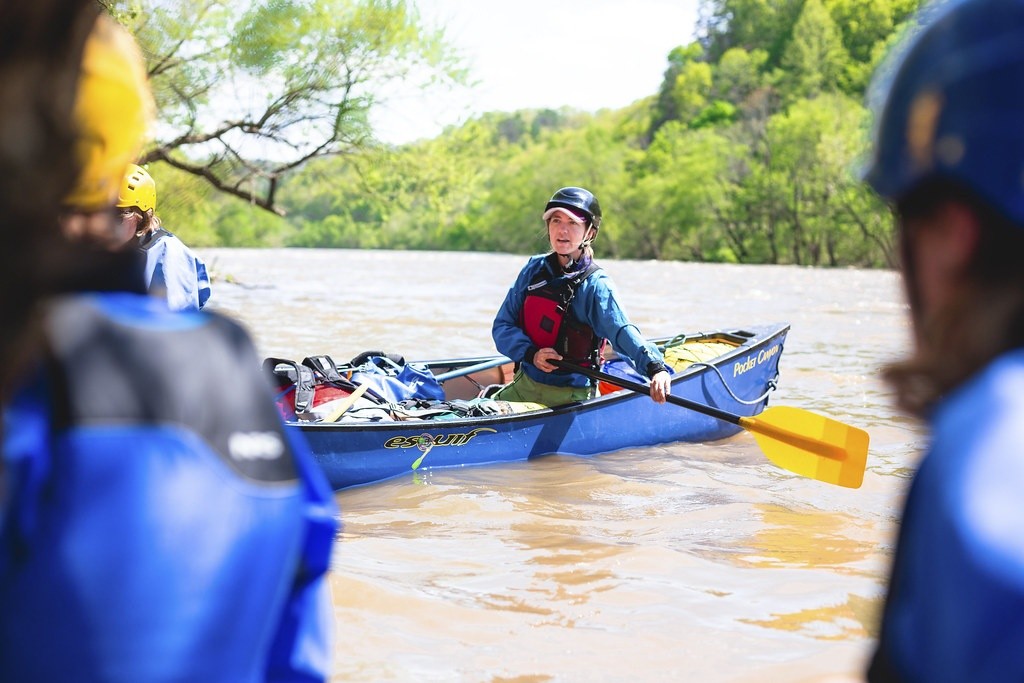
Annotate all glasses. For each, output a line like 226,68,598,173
111,207,136,227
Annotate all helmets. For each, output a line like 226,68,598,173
543,186,602,244
850,0,1024,219
56,14,150,208
114,163,157,222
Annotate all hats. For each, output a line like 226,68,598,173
543,205,591,225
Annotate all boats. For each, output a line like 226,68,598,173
250,321,792,498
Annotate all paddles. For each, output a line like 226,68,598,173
547,356,870,489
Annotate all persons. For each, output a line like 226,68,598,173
111,164,210,310
862,0,1024,683
489,187,671,407
0,0,336,683
